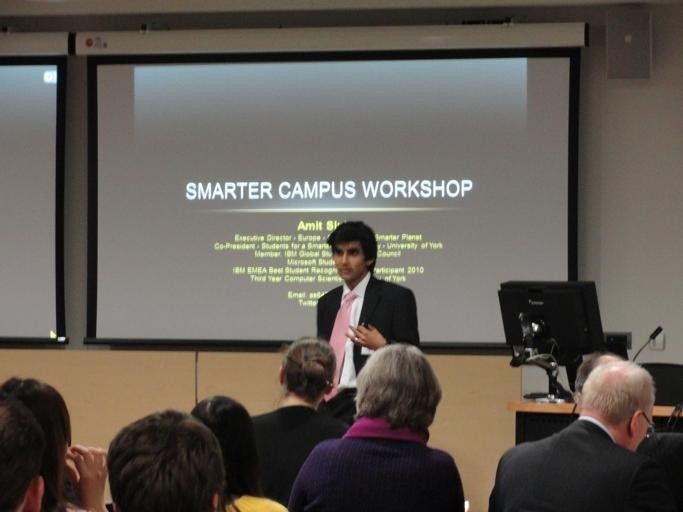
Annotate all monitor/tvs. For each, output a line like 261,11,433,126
497,281,605,402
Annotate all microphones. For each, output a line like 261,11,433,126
630,325,662,363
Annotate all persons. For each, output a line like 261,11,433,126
279,221,421,431
573,352,683,478
487,360,657,512
191,396,293,512
1,389,48,511
288,343,466,512
248,336,352,509
1,377,109,512
107,408,222,512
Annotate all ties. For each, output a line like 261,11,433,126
323,292,359,402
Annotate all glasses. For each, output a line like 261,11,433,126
641,411,656,438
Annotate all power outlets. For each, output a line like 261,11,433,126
648,333,664,351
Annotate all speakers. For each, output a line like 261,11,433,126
602,2,655,84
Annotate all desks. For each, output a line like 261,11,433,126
505,400,683,447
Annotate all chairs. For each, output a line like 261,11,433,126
637,362,683,406
